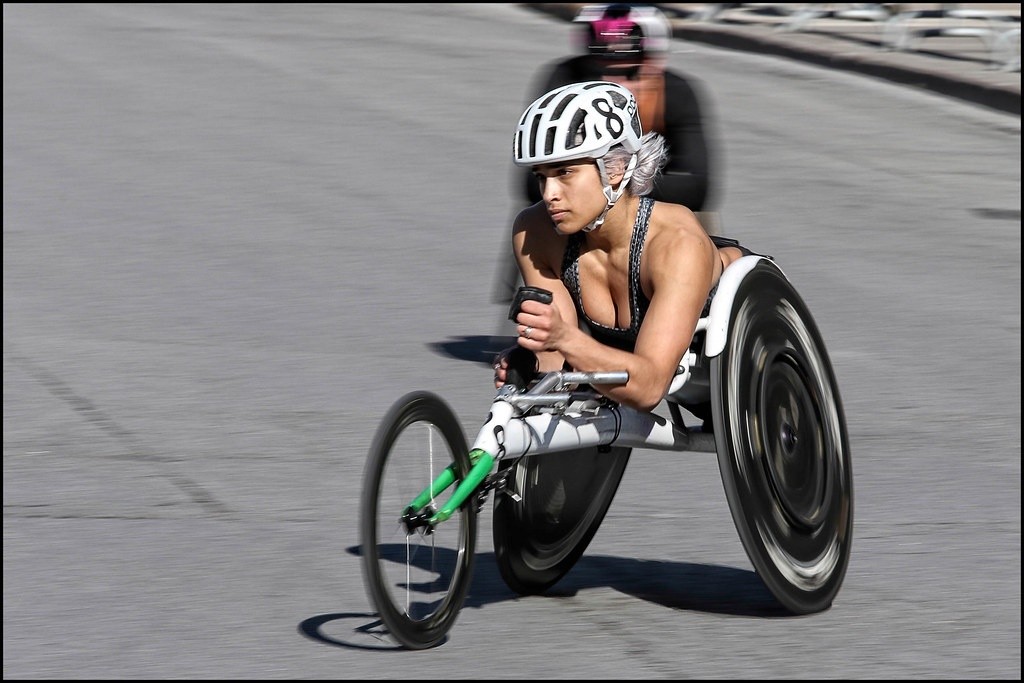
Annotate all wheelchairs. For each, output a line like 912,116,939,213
359,256,855,649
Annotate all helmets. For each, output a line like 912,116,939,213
512,79,641,165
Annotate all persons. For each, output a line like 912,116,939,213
493,4,751,412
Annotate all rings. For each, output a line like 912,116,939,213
522,326,532,338
